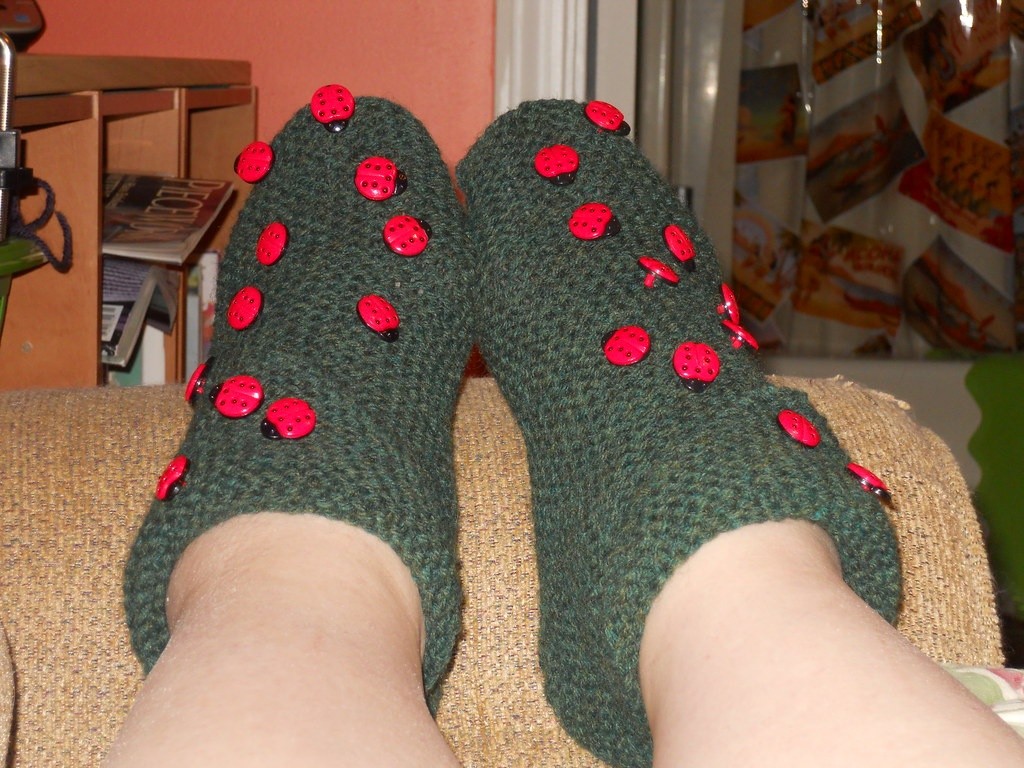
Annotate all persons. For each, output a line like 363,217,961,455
105,86,1024,767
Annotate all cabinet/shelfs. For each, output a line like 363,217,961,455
1,56,259,387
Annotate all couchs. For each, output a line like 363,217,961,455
0,377,1004,768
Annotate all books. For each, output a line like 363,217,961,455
103,169,234,266
102,251,220,386
